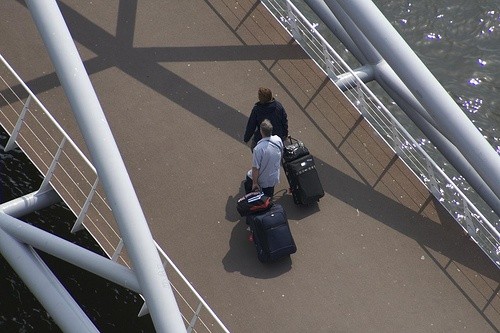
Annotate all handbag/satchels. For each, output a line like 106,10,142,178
236,188,272,216
284,135,309,161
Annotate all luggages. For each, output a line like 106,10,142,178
283,153,325,207
246,203,297,264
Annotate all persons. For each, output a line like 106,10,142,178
244,119,284,232
244,87,288,153
